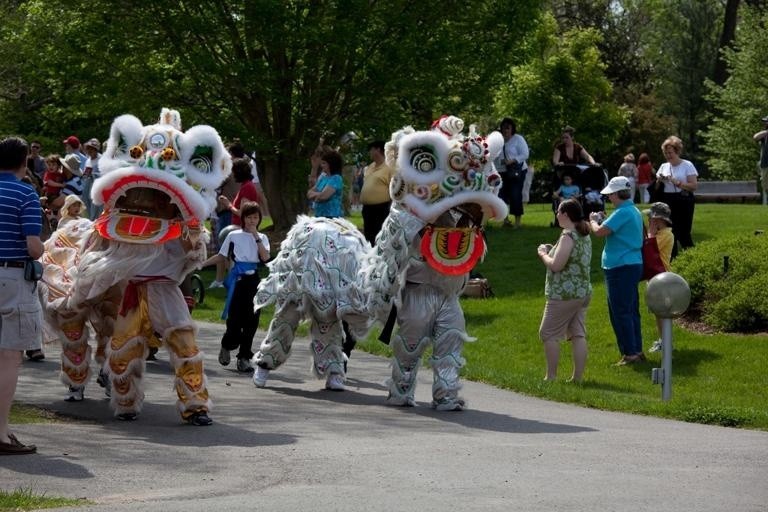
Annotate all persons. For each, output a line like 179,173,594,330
553,126,596,165
635,153,654,203
654,136,699,263
584,186,602,205
22,116,530,369
589,176,646,367
537,200,593,387
645,200,675,353
0,138,46,456
551,174,583,201
753,115,768,234
617,153,635,202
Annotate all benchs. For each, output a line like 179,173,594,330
692,180,760,197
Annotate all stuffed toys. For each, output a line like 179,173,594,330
251,301,347,391
383,281,466,411
102,283,213,425
58,302,111,403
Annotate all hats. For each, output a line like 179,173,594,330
641,201,674,226
63,135,80,148
82,137,101,151
59,153,84,177
599,176,632,195
60,194,87,216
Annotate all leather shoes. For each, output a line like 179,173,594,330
0,434,37,456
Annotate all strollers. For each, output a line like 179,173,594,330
551,163,580,228
581,163,609,225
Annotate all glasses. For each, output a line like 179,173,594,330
32,146,40,149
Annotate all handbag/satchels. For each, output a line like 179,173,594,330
506,162,523,179
642,237,666,281
24,260,43,282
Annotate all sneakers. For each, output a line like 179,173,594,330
648,338,665,354
326,374,345,393
253,364,269,388
218,345,230,365
614,352,646,367
236,357,254,373
189,410,213,427
63,385,84,402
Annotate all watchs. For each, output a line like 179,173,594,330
254,239,262,243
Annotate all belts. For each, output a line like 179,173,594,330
0,260,24,269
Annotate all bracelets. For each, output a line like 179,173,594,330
227,204,233,210
675,181,681,187
